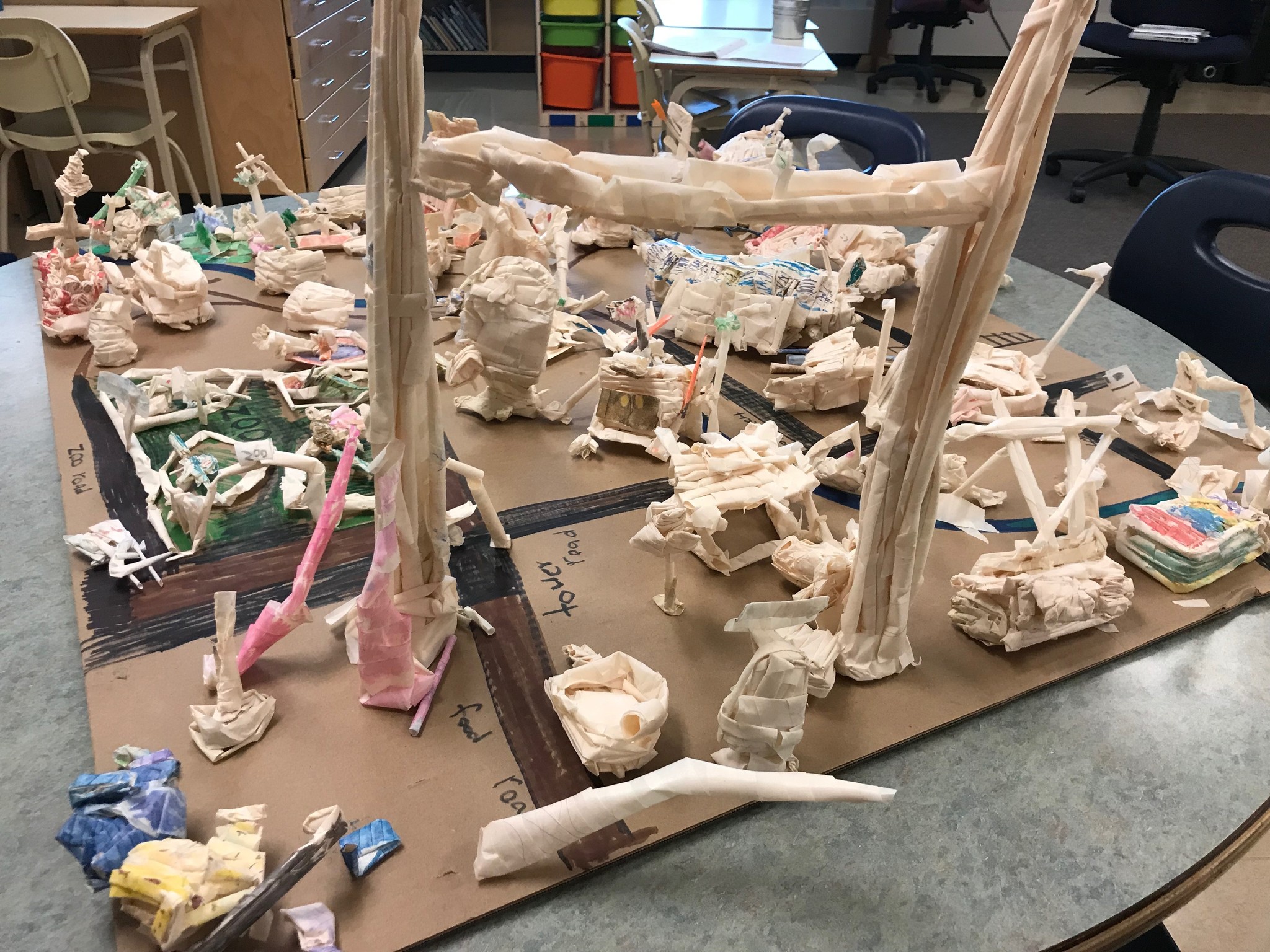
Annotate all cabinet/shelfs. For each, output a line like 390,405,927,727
419,0,537,73
14,0,372,195
534,0,643,127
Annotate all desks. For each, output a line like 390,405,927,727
653,0,820,32
648,26,839,153
1,191,1270,952
0,6,225,214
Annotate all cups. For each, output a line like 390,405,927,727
774,0,811,39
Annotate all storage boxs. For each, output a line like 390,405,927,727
541,0,639,110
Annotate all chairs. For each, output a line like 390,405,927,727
616,0,1270,413
0,18,204,254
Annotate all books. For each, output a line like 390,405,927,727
1129,24,1205,44
418,0,488,52
